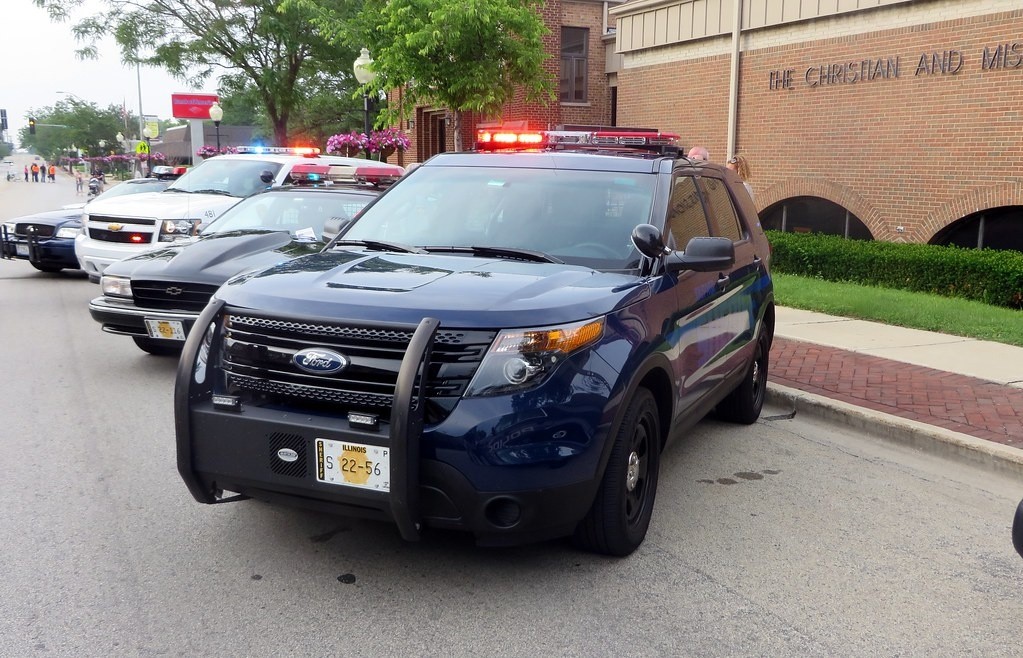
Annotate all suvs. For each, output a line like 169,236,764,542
172,127,776,559
74,146,401,281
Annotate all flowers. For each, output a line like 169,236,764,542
364,128,412,151
196,145,217,156
220,146,239,154
324,131,367,153
62,151,165,162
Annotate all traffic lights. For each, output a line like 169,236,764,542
28,117,35,134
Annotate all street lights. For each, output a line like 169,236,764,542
353,49,378,160
98,139,106,182
143,125,152,177
209,102,223,152
116,131,125,181
72,147,77,172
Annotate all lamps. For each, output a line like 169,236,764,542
409,116,415,129
445,115,451,126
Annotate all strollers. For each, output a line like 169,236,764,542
86,175,101,196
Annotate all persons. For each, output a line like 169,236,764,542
687,146,709,162
47,164,56,183
725,155,754,201
40,164,46,182
23,164,29,182
75,169,83,196
31,162,39,183
91,165,104,180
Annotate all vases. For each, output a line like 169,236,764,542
380,146,395,157
200,154,212,159
340,148,356,157
140,159,145,161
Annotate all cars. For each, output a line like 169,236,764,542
88,164,435,356
1,163,225,273
